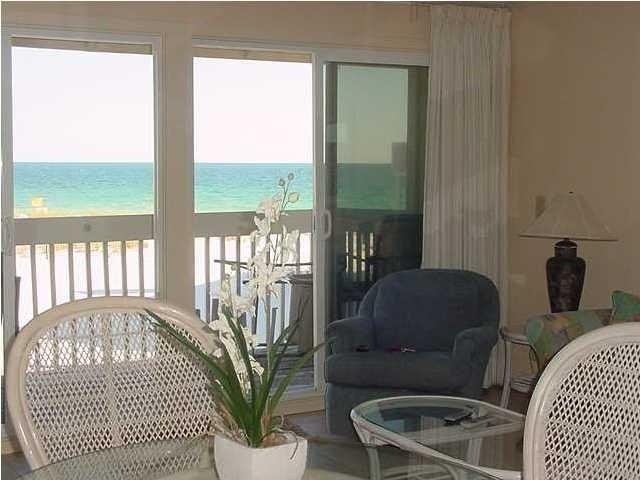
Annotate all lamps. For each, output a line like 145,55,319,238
521,192,618,313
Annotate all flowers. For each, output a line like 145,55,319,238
144,174,337,447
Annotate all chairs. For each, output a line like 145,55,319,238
324,269,500,436
333,211,424,322
4,296,243,479
523,321,640,480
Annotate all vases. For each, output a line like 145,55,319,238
214,430,308,479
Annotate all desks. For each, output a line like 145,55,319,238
500,322,538,409
12,437,492,480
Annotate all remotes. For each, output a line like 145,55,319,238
443,411,472,422
460,415,496,428
